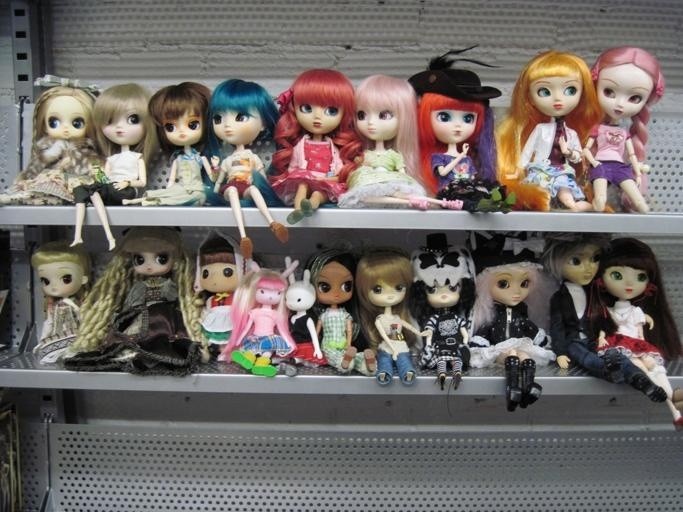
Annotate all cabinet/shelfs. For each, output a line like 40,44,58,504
0,200,682,400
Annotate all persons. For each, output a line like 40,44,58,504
15,45,664,250
13,232,682,434
13,45,682,432
